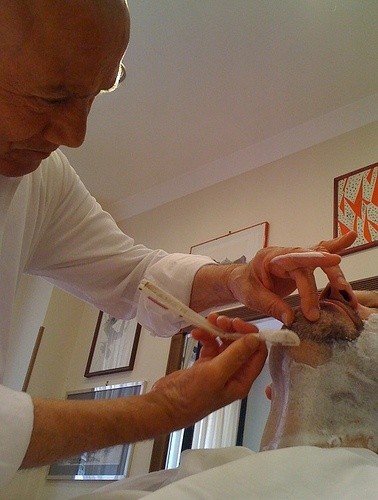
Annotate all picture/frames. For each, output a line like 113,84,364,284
333,163,378,257
84,310,142,378
45,381,147,480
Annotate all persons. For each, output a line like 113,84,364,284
0,0,363,484
69,281,378,499
